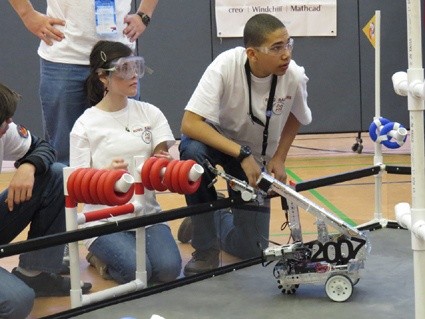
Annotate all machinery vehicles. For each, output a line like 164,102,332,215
251,169,372,302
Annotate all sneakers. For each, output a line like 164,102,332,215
184,249,219,277
12,243,92,297
178,217,192,243
85,252,112,280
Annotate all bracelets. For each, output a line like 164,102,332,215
136,11,151,26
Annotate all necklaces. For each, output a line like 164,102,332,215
112,105,131,132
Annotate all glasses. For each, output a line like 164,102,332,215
98,56,145,81
255,38,295,57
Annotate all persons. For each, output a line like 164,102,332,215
9,0,159,264
178,13,313,276
0,83,93,319
69,40,182,287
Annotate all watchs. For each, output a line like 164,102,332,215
237,145,252,162
